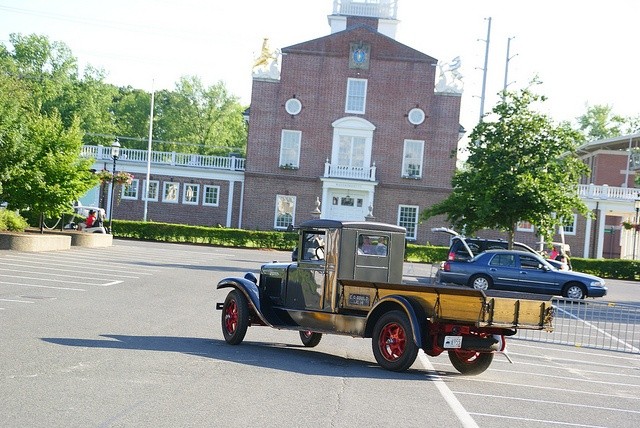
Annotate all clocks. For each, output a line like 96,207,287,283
353,50,366,63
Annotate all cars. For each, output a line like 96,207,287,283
431,227,608,299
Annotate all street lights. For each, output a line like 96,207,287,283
108,138,121,233
633,193,640,260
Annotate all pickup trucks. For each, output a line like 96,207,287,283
215,218,555,374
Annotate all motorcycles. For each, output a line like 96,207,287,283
64,206,107,234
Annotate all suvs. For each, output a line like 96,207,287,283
445,237,570,272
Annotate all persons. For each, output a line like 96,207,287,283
85,209,97,228
549,246,560,260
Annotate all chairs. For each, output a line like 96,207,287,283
85,218,99,228
555,255,564,261
